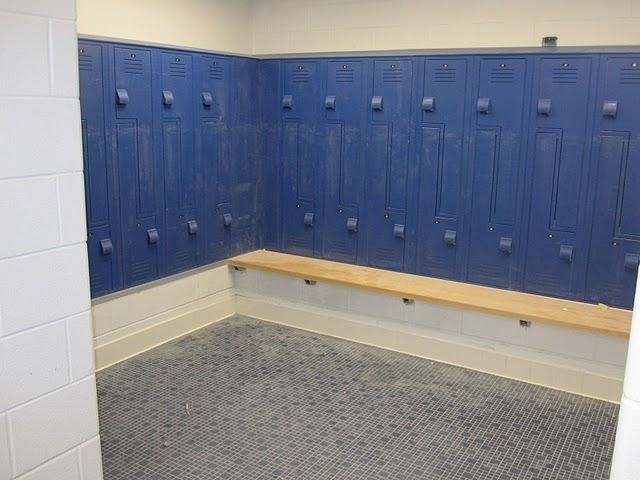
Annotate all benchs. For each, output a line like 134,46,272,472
227,250,632,340
